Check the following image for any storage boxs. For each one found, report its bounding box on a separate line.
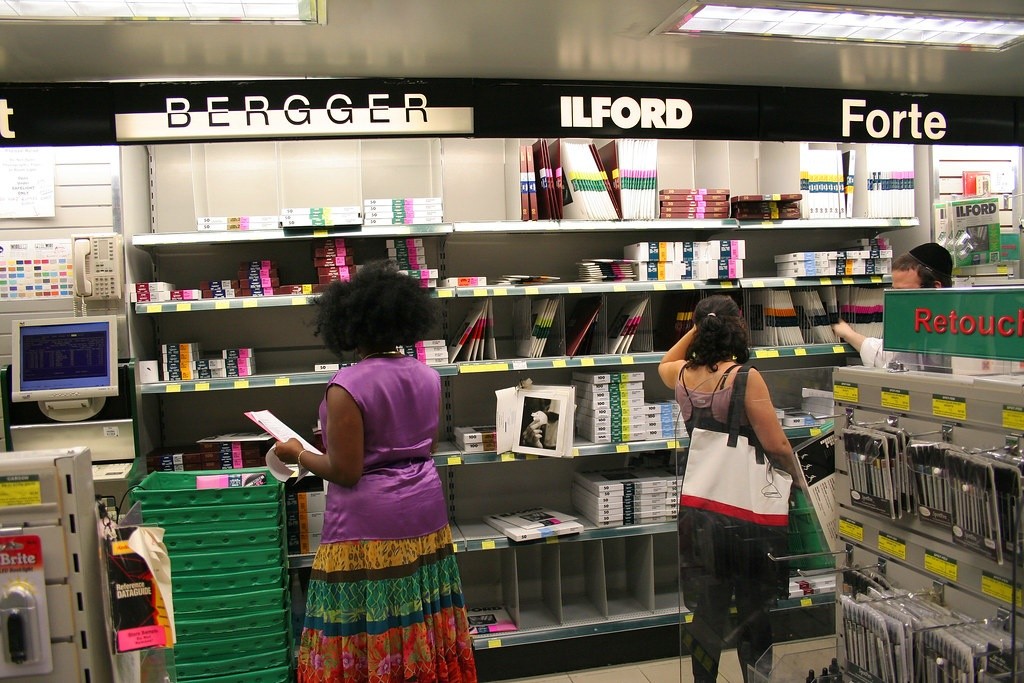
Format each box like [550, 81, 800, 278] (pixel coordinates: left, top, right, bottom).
[132, 464, 297, 682]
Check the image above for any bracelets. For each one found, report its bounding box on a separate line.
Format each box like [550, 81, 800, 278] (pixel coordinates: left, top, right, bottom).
[298, 450, 310, 468]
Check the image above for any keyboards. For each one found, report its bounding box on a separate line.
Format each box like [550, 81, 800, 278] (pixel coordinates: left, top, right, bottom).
[91, 463, 133, 479]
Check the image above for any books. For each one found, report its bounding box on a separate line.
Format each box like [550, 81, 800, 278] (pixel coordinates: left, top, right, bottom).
[448, 138, 916, 546]
[146, 432, 274, 474]
[102, 500, 178, 655]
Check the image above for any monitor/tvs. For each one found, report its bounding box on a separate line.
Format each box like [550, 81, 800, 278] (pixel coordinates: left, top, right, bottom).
[12, 315, 119, 402]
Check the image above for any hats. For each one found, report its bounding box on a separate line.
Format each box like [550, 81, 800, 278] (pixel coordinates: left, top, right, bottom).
[909, 243, 953, 277]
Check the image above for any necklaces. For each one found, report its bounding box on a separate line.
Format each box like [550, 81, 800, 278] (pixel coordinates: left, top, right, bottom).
[362, 351, 401, 360]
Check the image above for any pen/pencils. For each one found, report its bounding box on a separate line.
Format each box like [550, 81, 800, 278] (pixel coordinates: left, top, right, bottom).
[804, 564, 1024, 683]
[910, 438, 1024, 567]
[841, 420, 917, 521]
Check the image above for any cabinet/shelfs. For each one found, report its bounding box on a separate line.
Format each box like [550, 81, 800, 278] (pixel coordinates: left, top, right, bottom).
[811, 357, 1023, 683]
[116, 142, 918, 656]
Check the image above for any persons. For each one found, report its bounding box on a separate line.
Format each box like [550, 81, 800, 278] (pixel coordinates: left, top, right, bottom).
[657, 295, 811, 683]
[522, 419, 543, 448]
[272, 260, 476, 683]
[831, 241, 953, 373]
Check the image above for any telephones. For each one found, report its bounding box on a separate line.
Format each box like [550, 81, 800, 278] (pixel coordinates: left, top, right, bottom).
[70, 232, 124, 301]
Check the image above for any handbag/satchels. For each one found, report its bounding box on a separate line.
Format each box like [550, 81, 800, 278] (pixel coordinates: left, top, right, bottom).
[679, 364, 794, 527]
